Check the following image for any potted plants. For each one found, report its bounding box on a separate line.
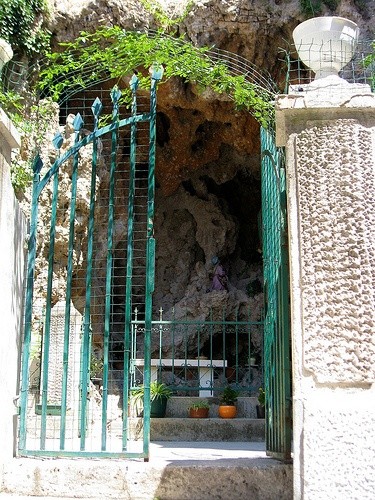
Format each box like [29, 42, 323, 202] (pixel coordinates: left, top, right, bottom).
[255, 387, 265, 419]
[246, 352, 255, 365]
[216, 385, 238, 419]
[185, 399, 211, 419]
[130, 379, 174, 418]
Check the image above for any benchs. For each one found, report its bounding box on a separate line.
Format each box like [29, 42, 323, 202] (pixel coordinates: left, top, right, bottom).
[129, 358, 228, 396]
